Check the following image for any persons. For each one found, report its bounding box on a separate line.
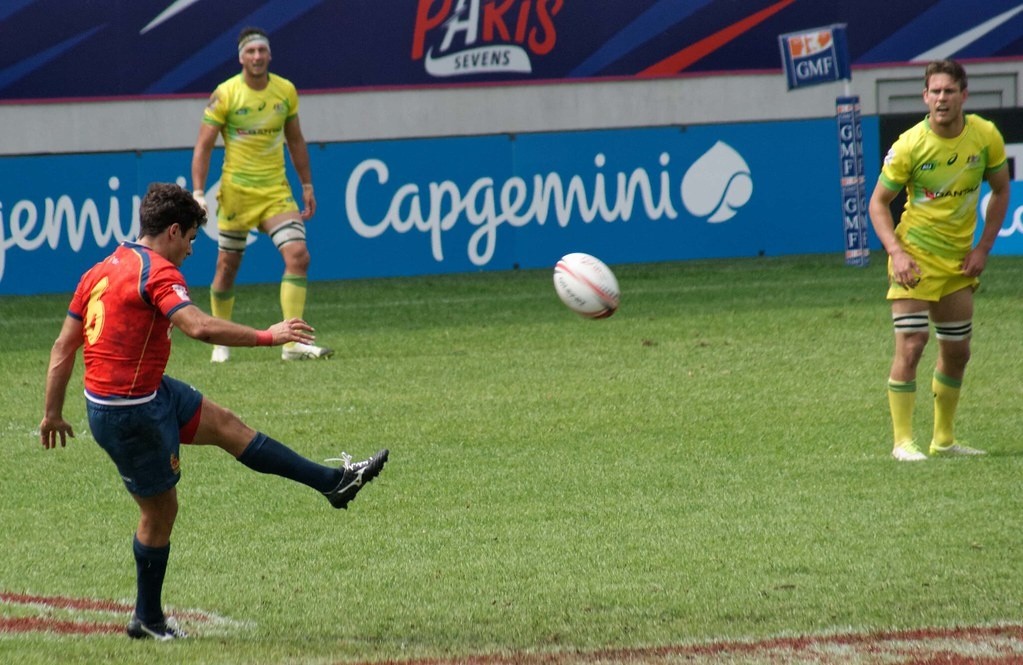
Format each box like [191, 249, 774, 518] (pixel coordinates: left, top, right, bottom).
[867, 59, 1011, 462]
[192, 26, 335, 363]
[39, 181, 392, 643]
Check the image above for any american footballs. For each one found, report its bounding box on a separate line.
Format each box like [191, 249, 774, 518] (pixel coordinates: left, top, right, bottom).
[553, 251, 620, 319]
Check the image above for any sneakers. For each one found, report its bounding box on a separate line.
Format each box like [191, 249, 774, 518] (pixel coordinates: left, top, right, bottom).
[929, 439, 986, 456]
[322, 449, 389, 511]
[893, 439, 927, 461]
[282, 342, 334, 359]
[127, 611, 188, 641]
[210, 346, 229, 362]
